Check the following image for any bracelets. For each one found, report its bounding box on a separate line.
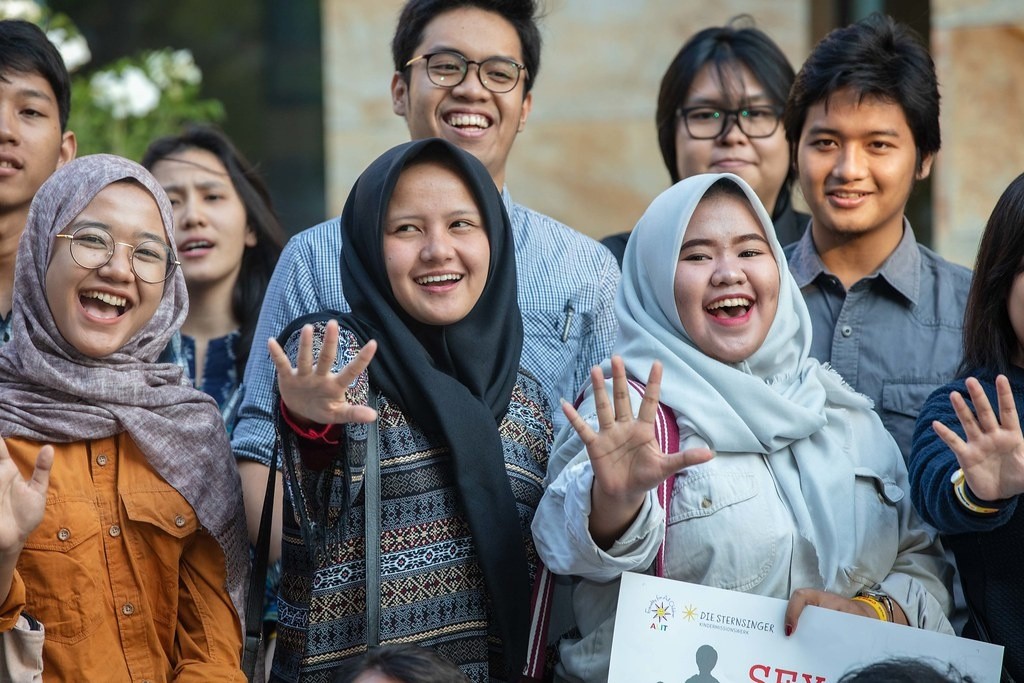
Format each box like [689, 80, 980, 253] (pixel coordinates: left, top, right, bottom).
[279, 400, 338, 445]
[852, 596, 887, 621]
[950, 468, 1000, 513]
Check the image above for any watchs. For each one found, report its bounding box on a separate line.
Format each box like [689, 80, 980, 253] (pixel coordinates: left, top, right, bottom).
[855, 589, 895, 622]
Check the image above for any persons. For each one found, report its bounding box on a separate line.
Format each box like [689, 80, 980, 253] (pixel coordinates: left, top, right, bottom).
[0, 0, 1024, 683]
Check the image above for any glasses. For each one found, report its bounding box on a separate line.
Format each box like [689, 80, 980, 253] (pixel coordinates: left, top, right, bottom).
[680, 106, 786, 140]
[403, 51, 530, 93]
[56, 225, 181, 284]
